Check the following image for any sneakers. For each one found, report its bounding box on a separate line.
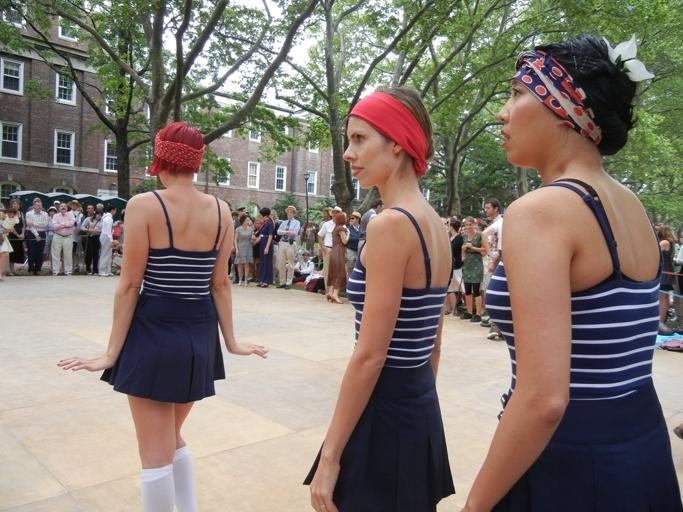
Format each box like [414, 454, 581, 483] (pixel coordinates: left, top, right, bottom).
[256, 282, 269, 288]
[86, 270, 92, 275]
[232, 279, 248, 286]
[75, 265, 80, 272]
[488, 331, 502, 339]
[277, 284, 290, 289]
[98, 271, 114, 276]
[8, 271, 21, 276]
[460, 311, 491, 327]
[29, 270, 41, 276]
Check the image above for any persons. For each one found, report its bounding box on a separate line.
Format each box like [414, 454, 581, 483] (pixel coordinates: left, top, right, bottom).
[57, 121, 268, 512]
[461, 35, 683, 510]
[440, 197, 505, 341]
[223, 200, 385, 304]
[1, 197, 126, 276]
[304, 87, 455, 511]
[653, 222, 682, 353]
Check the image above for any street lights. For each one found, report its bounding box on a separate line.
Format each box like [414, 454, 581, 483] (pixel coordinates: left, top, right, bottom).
[303, 171, 311, 223]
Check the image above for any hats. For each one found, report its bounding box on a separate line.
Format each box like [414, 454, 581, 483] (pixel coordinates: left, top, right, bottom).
[351, 211, 362, 218]
[324, 206, 334, 216]
[331, 205, 342, 215]
[285, 206, 297, 213]
[68, 200, 81, 208]
[54, 201, 60, 205]
[47, 206, 58, 213]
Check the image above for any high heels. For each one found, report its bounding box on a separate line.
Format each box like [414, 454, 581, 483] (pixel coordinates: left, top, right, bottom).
[326, 294, 343, 304]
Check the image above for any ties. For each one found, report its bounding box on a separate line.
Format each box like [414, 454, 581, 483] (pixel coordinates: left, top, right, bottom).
[284, 220, 291, 240]
